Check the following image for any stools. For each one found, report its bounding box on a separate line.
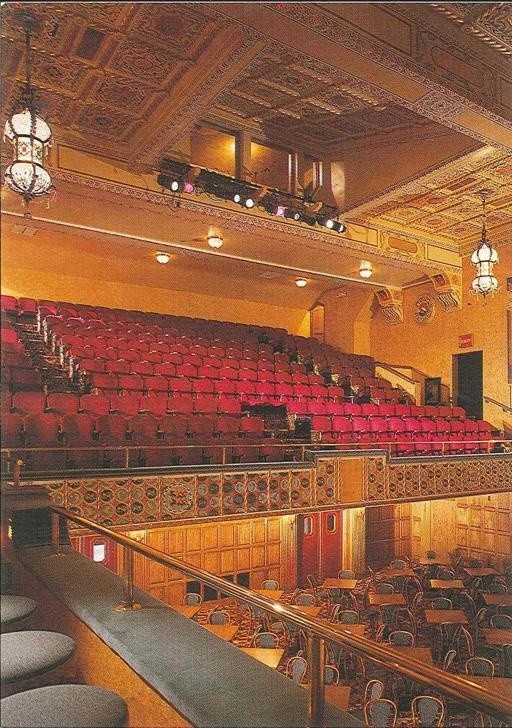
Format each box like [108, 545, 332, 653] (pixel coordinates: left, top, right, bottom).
[1, 682, 131, 728]
[2, 592, 39, 628]
[1, 629, 79, 687]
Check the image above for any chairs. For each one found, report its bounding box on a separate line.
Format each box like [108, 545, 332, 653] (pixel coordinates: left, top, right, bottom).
[164, 551, 511, 727]
[0, 288, 512, 466]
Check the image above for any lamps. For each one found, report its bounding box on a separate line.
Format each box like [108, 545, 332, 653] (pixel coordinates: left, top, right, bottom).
[155, 157, 371, 289]
[468, 185, 498, 300]
[3, 13, 54, 202]
